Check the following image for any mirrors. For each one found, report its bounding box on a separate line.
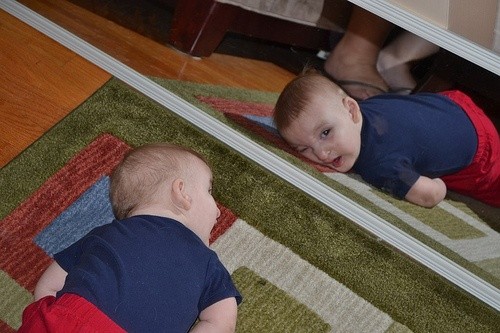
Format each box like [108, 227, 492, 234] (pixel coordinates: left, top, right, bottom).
[14, 0, 500, 293]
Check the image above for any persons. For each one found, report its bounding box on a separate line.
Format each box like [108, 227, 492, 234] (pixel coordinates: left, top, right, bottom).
[321, 5, 442, 102]
[274, 71, 499, 208]
[15, 142, 243, 333]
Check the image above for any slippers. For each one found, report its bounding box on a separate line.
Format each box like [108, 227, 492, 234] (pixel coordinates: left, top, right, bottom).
[322, 61, 392, 103]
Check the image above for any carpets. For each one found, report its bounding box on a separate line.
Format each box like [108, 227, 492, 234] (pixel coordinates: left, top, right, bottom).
[1, 76, 500, 332]
[147, 74, 499, 290]
